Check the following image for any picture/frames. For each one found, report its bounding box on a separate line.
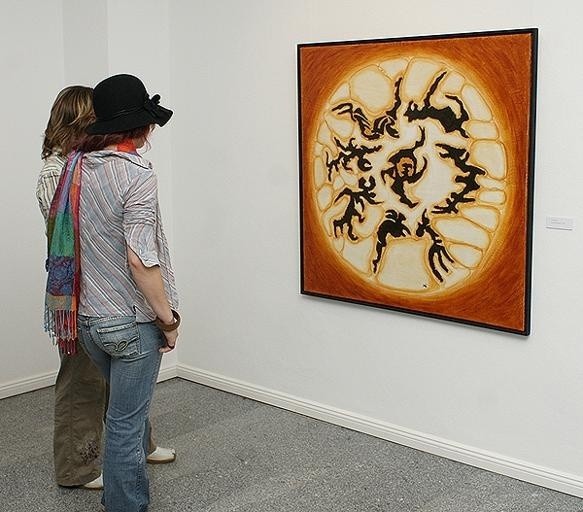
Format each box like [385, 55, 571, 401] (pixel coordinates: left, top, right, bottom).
[293, 29, 539, 341]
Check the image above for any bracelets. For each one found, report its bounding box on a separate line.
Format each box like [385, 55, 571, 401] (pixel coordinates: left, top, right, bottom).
[154, 311, 181, 332]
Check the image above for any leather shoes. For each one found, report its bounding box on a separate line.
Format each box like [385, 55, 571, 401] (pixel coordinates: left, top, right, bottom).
[81, 472, 104, 488]
[144, 445, 175, 463]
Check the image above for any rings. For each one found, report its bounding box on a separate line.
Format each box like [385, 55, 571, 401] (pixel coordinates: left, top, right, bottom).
[168, 344, 175, 349]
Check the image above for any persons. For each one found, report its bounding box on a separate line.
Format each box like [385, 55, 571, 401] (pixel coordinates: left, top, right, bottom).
[36, 85, 176, 490]
[66, 74, 181, 512]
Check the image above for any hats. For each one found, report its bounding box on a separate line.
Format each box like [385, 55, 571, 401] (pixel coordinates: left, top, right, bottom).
[85, 74, 173, 134]
